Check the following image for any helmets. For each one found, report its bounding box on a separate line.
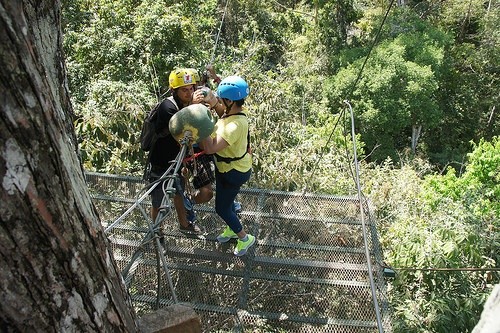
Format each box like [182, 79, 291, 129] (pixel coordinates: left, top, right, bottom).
[216, 76, 249, 100]
[169, 68, 196, 89]
[187, 68, 200, 81]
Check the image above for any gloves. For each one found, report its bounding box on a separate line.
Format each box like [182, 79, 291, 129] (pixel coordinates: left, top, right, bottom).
[201, 87, 218, 108]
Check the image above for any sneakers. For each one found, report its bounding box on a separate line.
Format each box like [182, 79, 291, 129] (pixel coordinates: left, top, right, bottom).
[216, 225, 239, 242]
[234, 234, 256, 256]
[180, 223, 205, 235]
[185, 194, 195, 221]
[159, 229, 166, 245]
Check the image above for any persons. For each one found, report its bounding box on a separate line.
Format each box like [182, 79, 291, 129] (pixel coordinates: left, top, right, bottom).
[187, 64, 222, 206]
[201, 76, 256, 256]
[143, 68, 205, 255]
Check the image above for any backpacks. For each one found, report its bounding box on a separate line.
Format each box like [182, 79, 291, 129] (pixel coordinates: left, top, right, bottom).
[139, 97, 180, 152]
